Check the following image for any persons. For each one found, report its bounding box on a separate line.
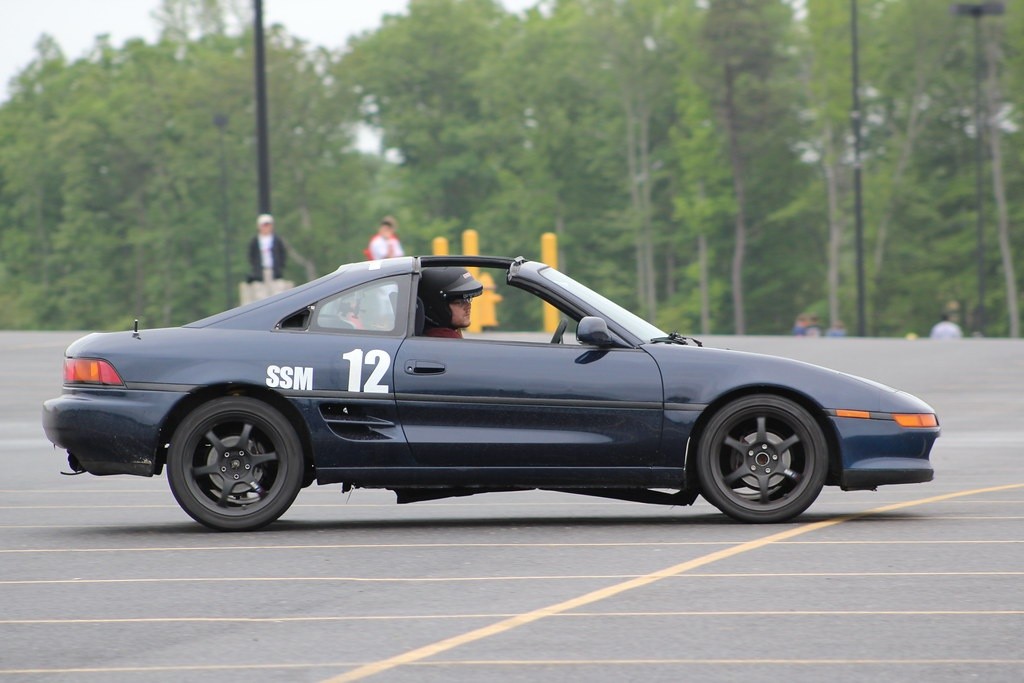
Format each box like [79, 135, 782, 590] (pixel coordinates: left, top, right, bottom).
[930, 312, 961, 340]
[793, 313, 848, 337]
[417, 267, 483, 338]
[365, 215, 405, 260]
[243, 214, 286, 300]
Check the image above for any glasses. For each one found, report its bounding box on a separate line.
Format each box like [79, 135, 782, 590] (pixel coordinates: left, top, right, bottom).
[450, 298, 473, 305]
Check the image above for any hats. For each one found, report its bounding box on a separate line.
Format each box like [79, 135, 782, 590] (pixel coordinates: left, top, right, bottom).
[257, 214, 273, 224]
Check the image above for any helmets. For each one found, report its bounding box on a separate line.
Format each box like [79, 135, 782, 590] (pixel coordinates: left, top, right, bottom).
[417, 266, 483, 328]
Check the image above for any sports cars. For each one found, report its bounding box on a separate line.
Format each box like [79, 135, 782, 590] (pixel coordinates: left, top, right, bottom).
[40, 254, 943, 535]
